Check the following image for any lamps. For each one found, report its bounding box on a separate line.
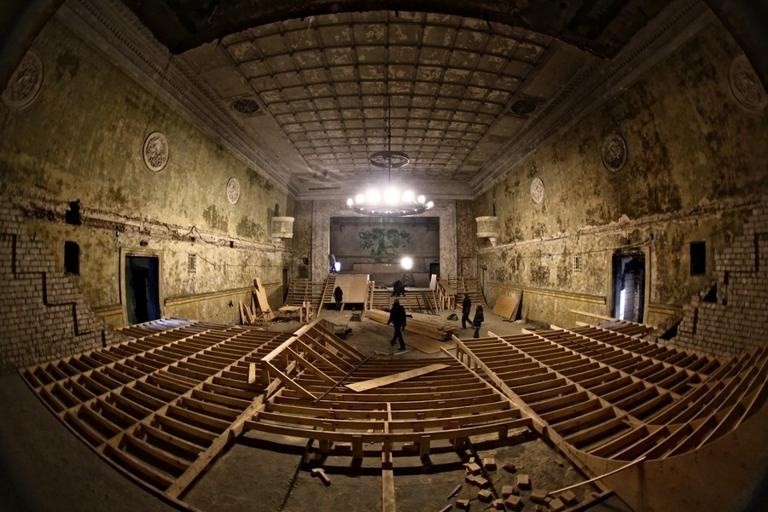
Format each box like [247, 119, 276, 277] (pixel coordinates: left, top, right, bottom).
[347, 97, 434, 218]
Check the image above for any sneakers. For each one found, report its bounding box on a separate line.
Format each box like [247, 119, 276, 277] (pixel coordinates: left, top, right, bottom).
[397, 348, 406, 350]
[390, 340, 396, 343]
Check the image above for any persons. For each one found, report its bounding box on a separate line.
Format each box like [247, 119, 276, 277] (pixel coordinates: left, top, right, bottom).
[391, 279, 406, 297]
[462, 292, 484, 338]
[328, 253, 336, 275]
[333, 286, 343, 311]
[386, 298, 407, 351]
